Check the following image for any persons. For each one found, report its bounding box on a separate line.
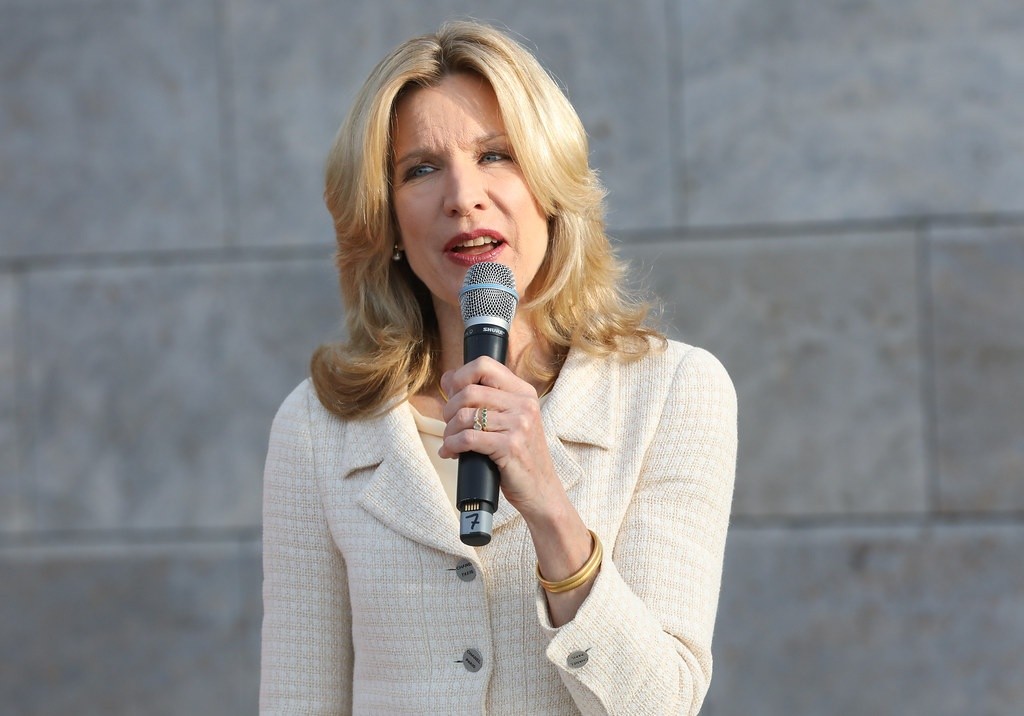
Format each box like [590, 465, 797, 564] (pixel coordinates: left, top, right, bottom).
[261, 23, 738, 716]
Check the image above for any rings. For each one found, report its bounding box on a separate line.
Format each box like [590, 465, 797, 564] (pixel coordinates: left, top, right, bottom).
[482, 408, 487, 431]
[474, 408, 482, 431]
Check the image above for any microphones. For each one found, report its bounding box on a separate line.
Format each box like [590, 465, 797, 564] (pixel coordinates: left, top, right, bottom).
[458, 263, 519, 549]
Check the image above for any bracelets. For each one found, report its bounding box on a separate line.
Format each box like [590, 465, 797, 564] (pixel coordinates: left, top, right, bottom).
[536, 532, 603, 594]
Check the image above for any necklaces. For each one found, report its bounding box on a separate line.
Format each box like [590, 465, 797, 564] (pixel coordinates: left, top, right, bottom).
[437, 381, 555, 403]
